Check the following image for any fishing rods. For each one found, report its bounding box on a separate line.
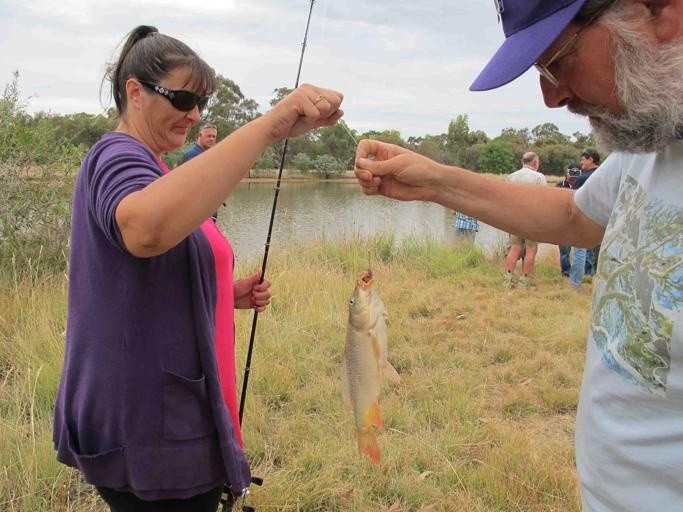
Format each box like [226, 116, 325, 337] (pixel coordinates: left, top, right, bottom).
[220, 0, 376, 512]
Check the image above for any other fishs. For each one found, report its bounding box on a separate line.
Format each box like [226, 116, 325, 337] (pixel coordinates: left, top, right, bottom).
[343, 268, 402, 468]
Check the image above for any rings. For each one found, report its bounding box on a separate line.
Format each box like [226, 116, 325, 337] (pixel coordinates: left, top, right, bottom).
[313, 94, 324, 106]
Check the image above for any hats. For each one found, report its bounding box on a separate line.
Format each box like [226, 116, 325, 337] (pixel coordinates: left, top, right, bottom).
[469, 1, 584, 91]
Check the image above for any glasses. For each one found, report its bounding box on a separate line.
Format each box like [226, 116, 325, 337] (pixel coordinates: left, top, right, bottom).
[136, 80, 209, 113]
[533, 1, 611, 86]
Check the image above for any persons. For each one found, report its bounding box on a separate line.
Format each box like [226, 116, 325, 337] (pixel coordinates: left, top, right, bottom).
[46, 24, 346, 510]
[499, 151, 550, 294]
[181, 123, 218, 226]
[565, 149, 604, 288]
[352, 1, 681, 512]
[447, 207, 479, 246]
[552, 162, 593, 280]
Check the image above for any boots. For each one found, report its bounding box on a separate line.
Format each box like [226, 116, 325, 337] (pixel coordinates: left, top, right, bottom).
[502, 273, 514, 289]
[518, 276, 537, 291]
